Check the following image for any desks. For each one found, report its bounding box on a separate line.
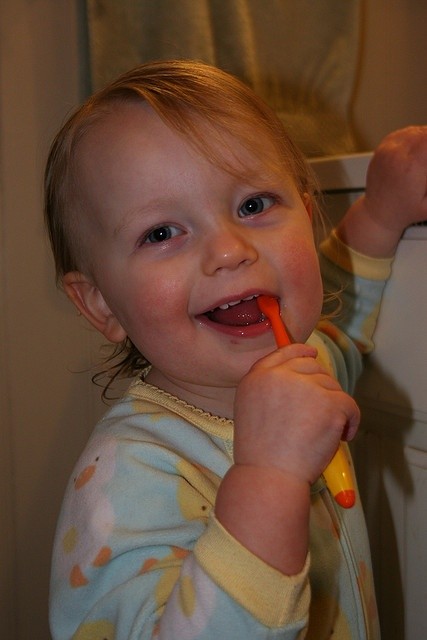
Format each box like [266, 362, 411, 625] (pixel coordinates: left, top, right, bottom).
[287, 149, 425, 639]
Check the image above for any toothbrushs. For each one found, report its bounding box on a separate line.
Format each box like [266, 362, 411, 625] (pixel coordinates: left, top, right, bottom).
[257, 294, 356, 508]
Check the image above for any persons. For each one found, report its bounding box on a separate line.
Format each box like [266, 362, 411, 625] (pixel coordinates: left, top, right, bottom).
[44, 57, 427, 639]
